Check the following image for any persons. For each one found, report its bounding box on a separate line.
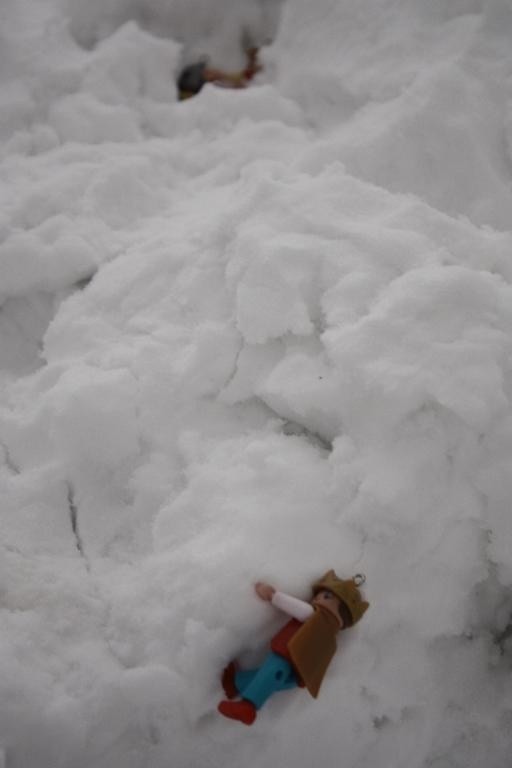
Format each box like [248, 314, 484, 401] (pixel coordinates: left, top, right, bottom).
[213, 568, 371, 726]
